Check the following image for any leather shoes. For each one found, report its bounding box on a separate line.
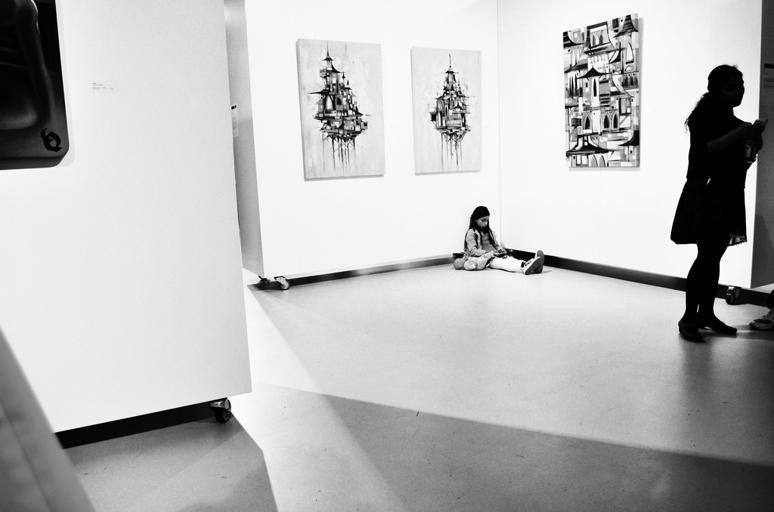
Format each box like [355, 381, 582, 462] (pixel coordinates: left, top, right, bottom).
[678, 313, 737, 342]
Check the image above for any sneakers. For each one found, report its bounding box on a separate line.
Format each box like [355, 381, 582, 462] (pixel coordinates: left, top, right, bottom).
[522, 250, 544, 275]
[748, 311, 774, 332]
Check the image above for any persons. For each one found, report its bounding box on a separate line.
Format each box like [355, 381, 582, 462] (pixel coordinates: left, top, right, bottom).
[462, 206, 545, 275]
[748, 286, 774, 333]
[667, 63, 766, 342]
